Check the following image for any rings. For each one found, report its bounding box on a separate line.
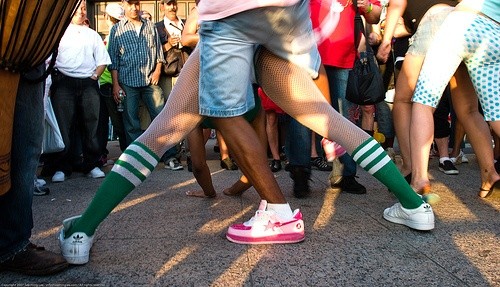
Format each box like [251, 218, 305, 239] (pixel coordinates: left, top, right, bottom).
[361, 0, 364, 4]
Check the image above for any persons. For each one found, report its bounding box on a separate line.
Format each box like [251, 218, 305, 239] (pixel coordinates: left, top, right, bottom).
[58, 41, 436, 259]
[32, 0, 500, 204]
[0, 63, 70, 275]
[195, 0, 347, 246]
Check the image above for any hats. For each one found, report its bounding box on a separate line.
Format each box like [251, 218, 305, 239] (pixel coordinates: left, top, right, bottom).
[162, 0, 177, 4]
[106, 3, 124, 20]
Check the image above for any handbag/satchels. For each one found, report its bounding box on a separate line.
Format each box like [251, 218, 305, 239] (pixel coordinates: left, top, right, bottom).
[40, 97, 65, 154]
[163, 46, 185, 78]
[345, 49, 386, 105]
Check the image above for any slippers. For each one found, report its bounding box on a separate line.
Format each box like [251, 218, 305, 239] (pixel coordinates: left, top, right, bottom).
[478, 180, 499, 198]
[387, 173, 412, 192]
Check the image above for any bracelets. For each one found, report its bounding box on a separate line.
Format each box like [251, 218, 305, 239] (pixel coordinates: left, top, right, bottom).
[364, 2, 372, 13]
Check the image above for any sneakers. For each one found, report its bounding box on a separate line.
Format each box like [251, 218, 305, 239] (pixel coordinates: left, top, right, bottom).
[34, 178, 46, 185]
[292, 174, 309, 197]
[321, 139, 346, 161]
[34, 183, 49, 195]
[461, 153, 468, 162]
[285, 163, 291, 171]
[310, 156, 333, 171]
[59, 214, 98, 264]
[163, 159, 182, 171]
[220, 157, 238, 171]
[87, 167, 105, 178]
[187, 156, 193, 172]
[330, 172, 367, 195]
[52, 171, 65, 181]
[225, 198, 306, 244]
[384, 201, 435, 232]
[270, 158, 282, 172]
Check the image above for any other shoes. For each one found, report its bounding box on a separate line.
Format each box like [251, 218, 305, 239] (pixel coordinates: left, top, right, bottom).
[0, 243, 68, 276]
[429, 149, 439, 159]
[214, 145, 220, 152]
[439, 159, 459, 175]
